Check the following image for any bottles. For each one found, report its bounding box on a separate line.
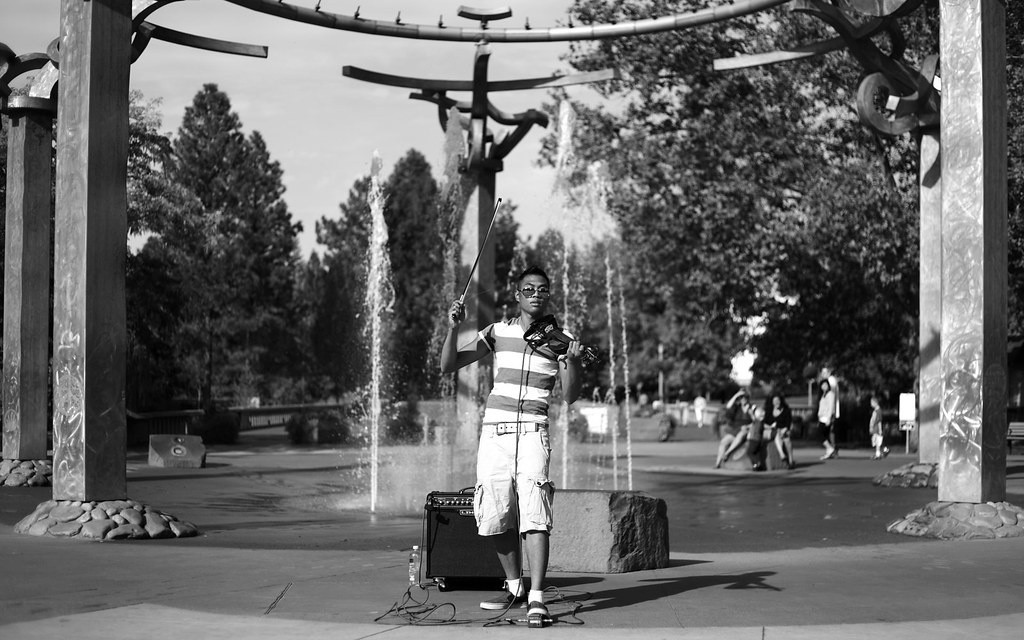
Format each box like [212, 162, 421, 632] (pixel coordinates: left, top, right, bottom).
[408, 545, 421, 586]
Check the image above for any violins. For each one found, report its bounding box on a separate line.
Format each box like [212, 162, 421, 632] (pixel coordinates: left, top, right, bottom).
[524, 314, 606, 368]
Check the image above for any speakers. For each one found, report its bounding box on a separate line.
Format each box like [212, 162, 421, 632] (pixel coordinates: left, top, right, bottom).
[426, 490, 509, 579]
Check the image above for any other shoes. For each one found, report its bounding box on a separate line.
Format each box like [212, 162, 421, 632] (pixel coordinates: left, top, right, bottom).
[820, 448, 835, 460]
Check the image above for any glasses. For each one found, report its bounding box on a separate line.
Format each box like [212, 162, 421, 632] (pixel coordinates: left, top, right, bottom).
[516, 287, 549, 299]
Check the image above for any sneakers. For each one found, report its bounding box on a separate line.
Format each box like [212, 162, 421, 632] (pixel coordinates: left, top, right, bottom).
[526, 601, 549, 620]
[479, 591, 528, 609]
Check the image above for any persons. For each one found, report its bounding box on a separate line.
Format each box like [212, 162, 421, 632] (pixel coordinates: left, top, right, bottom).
[440, 266, 583, 628]
[633, 363, 891, 473]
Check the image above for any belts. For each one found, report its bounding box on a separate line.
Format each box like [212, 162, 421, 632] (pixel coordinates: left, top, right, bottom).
[495, 423, 539, 435]
